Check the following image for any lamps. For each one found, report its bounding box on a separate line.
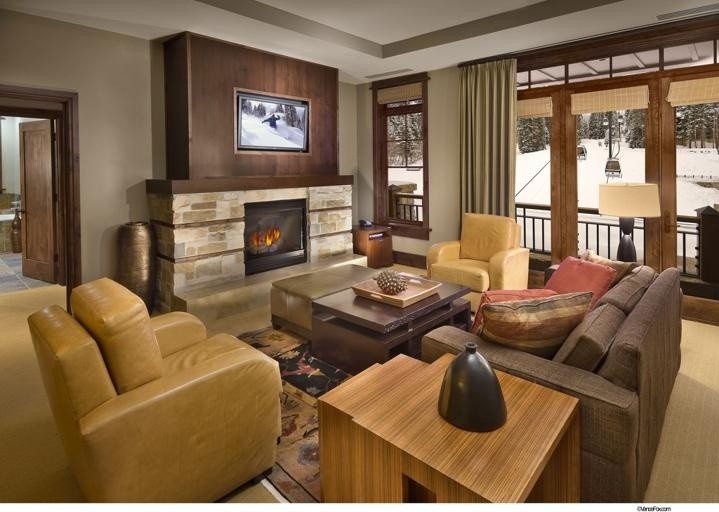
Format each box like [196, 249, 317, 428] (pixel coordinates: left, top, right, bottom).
[598, 183, 661, 262]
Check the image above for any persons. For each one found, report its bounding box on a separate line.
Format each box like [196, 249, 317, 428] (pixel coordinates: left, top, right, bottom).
[261, 114, 281, 130]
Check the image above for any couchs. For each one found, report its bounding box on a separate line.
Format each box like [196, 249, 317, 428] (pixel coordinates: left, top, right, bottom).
[419, 263, 684, 503]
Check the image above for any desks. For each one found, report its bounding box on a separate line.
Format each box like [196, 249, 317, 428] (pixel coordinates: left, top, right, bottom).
[353, 224, 393, 268]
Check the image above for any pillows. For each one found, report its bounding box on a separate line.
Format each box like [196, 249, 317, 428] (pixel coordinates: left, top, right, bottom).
[470, 255, 660, 372]
[459, 213, 516, 262]
[70, 276, 165, 396]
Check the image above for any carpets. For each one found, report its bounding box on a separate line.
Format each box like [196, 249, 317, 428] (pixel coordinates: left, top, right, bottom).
[234, 325, 356, 502]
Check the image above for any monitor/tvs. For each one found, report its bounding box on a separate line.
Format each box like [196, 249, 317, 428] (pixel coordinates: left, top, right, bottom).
[237, 94, 309, 151]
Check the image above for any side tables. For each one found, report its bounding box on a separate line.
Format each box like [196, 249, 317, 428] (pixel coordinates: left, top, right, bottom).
[317, 351, 582, 502]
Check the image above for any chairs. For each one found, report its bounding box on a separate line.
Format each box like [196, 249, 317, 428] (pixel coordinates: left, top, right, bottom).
[26, 279, 283, 502]
[425, 211, 530, 315]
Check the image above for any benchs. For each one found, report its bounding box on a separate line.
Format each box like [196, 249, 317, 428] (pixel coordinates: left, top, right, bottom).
[270, 264, 383, 342]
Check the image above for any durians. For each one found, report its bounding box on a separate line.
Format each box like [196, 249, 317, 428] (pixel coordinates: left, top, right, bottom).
[376, 270, 408, 294]
